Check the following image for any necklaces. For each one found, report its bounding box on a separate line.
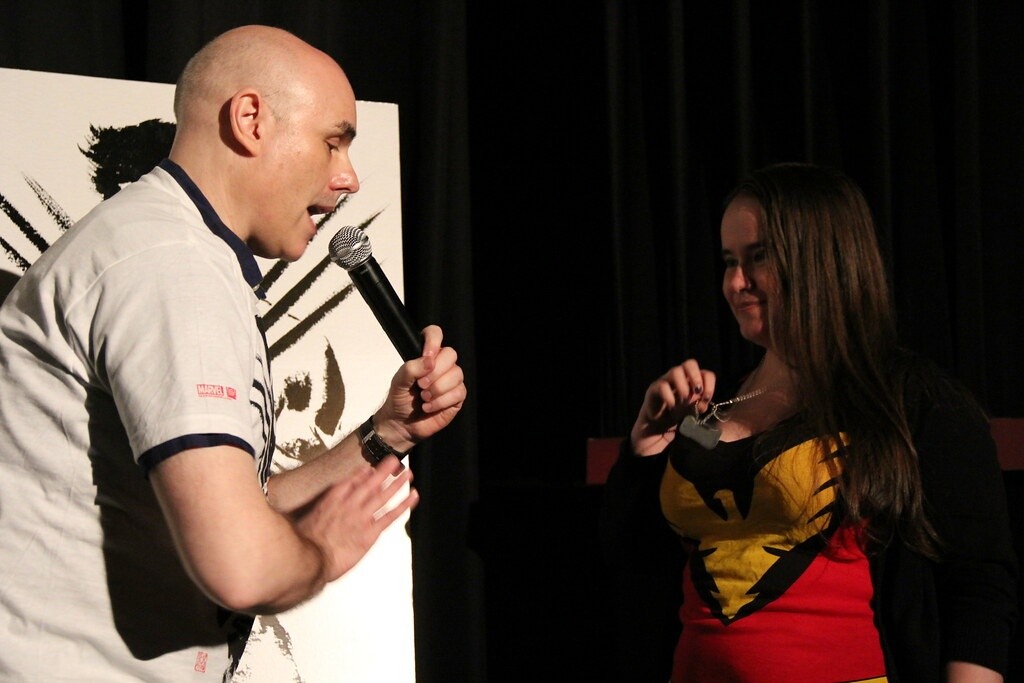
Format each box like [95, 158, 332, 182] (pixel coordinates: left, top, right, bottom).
[679, 354, 779, 449]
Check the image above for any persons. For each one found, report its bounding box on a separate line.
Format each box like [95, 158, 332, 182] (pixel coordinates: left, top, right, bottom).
[0, 25, 466, 683]
[604, 162, 1001, 683]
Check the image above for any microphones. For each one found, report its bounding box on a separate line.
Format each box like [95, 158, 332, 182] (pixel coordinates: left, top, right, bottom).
[328, 227, 425, 363]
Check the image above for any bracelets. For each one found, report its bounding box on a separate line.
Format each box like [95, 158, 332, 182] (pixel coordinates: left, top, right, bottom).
[358, 414, 411, 477]
